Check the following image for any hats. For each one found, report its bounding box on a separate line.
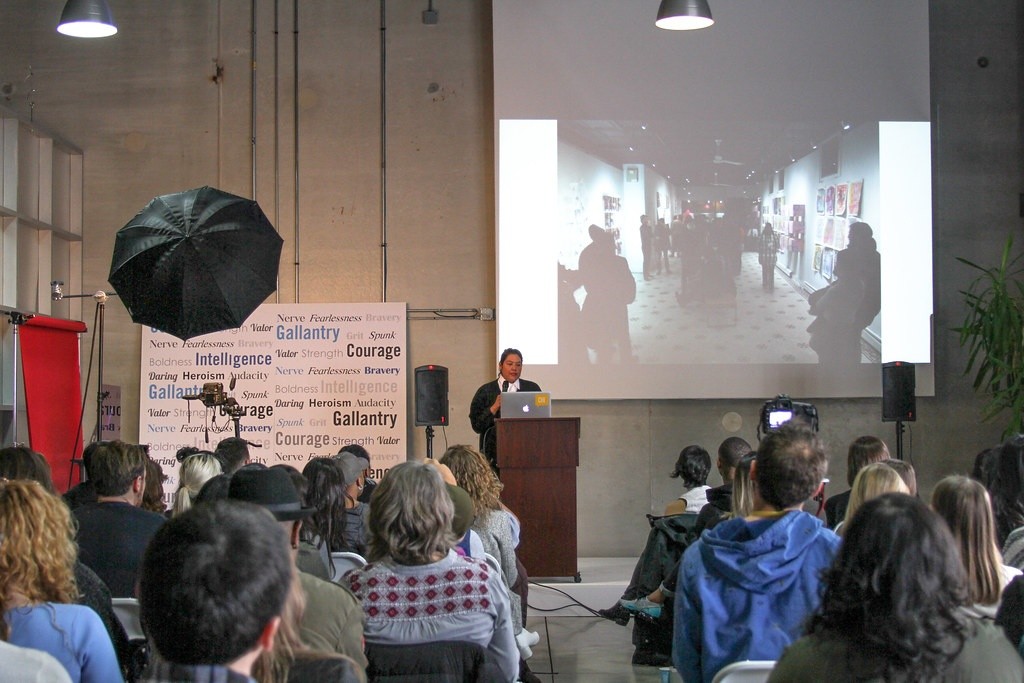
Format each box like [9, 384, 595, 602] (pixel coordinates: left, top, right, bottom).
[227, 466, 319, 522]
[331, 451, 368, 485]
[658, 217, 665, 222]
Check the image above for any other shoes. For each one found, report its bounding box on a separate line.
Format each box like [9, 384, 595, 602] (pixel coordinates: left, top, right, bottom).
[666, 269, 672, 272]
[644, 276, 651, 280]
[657, 270, 661, 274]
[599, 601, 630, 626]
[620, 598, 666, 623]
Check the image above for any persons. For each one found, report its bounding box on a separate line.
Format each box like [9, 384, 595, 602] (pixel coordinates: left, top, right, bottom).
[133, 437, 379, 683]
[338, 445, 542, 683]
[599, 416, 1024, 683]
[468, 348, 541, 480]
[558, 213, 881, 362]
[0, 448, 128, 683]
[60, 441, 169, 598]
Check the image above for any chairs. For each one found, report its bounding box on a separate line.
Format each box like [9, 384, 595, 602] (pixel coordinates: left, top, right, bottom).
[631, 514, 700, 668]
[366, 639, 510, 683]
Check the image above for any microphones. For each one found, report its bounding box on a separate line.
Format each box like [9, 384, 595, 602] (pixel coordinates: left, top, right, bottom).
[502, 381, 509, 393]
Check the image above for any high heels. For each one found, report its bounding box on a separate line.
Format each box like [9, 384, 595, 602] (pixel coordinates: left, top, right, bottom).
[515, 628, 539, 660]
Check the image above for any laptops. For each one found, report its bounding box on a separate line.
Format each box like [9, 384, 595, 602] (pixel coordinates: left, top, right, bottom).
[501, 391, 551, 417]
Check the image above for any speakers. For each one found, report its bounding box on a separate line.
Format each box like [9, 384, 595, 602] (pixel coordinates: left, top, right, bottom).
[414, 365, 450, 426]
[882, 361, 917, 421]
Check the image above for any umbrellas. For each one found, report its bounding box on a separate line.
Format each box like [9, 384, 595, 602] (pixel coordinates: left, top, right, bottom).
[108, 184, 284, 341]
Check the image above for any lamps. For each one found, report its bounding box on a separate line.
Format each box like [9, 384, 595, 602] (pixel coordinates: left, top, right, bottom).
[655, 0, 715, 31]
[56, 0, 118, 38]
